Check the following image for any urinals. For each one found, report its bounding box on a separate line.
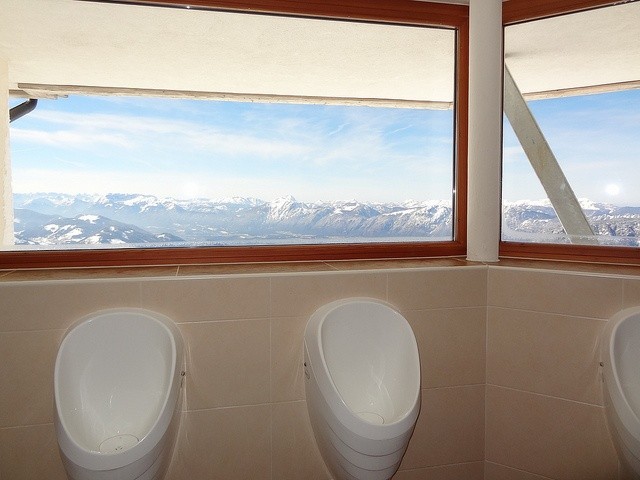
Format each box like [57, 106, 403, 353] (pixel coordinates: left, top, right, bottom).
[50, 308, 184, 477]
[600, 306, 639, 475]
[303, 296, 424, 479]
